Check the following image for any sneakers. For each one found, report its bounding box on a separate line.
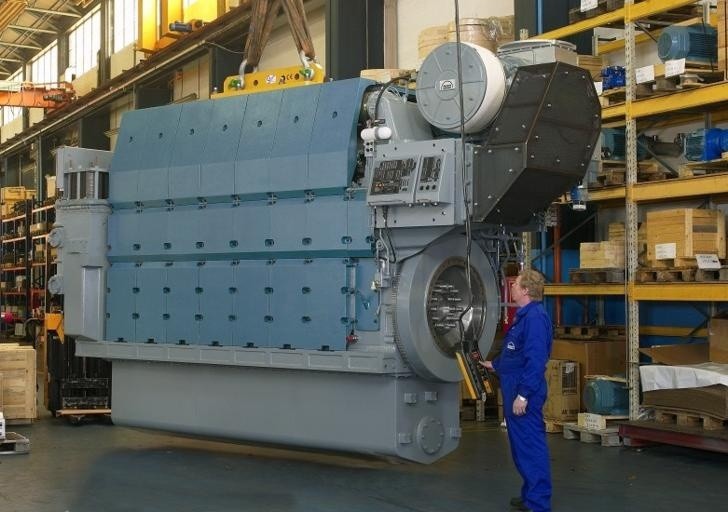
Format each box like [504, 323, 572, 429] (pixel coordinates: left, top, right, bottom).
[511, 498, 554, 511]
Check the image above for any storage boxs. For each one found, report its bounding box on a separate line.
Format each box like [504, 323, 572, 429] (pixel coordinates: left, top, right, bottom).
[0, 344, 38, 420]
[503, 208, 727, 421]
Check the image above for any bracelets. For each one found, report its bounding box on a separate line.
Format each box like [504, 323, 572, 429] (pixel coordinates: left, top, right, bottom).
[518, 393, 527, 401]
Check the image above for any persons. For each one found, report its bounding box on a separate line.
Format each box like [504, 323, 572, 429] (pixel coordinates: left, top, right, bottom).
[478, 269, 556, 512]
